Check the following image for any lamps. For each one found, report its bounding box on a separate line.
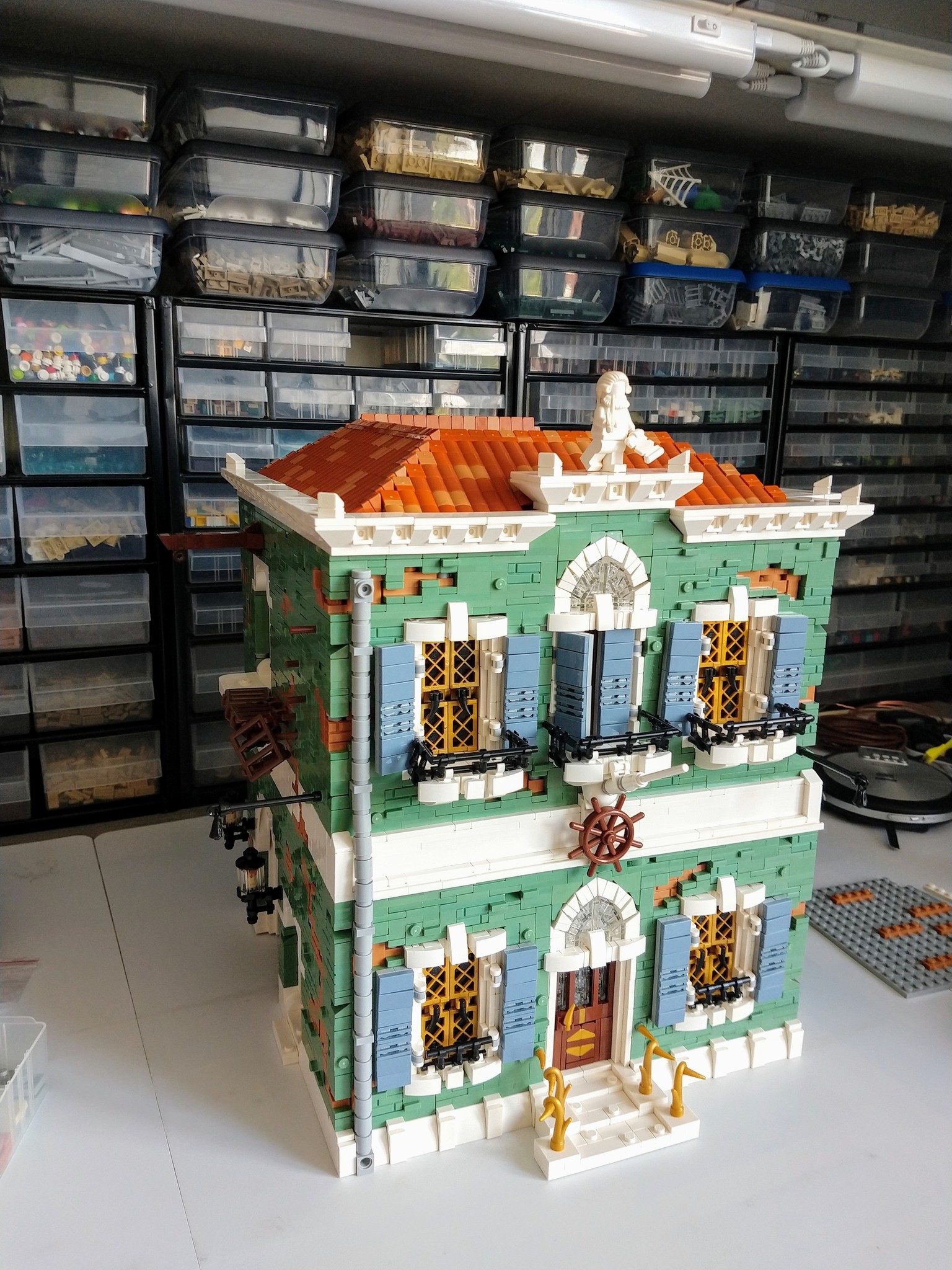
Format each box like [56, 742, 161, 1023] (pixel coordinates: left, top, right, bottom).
[170, 1, 952, 148]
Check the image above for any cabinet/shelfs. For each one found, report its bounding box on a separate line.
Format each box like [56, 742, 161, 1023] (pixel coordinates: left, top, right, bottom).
[3, 71, 950, 839]
